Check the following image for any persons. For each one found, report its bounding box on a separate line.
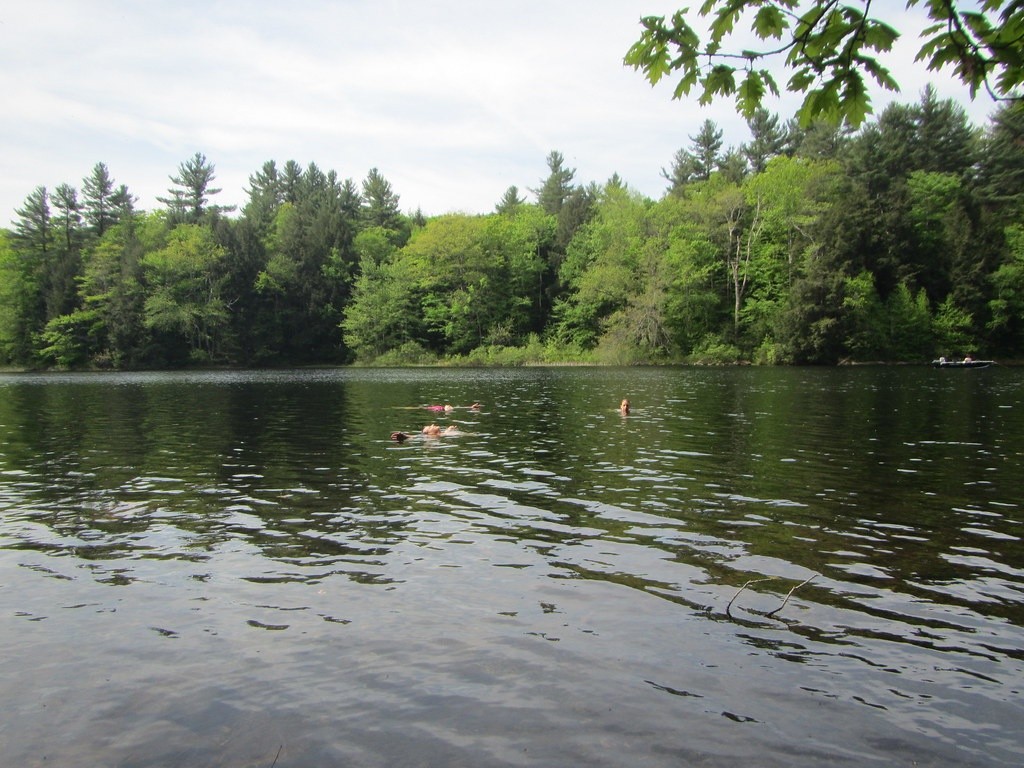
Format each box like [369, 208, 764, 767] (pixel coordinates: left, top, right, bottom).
[621, 399, 630, 416]
[391, 424, 458, 441]
[444, 402, 480, 412]
